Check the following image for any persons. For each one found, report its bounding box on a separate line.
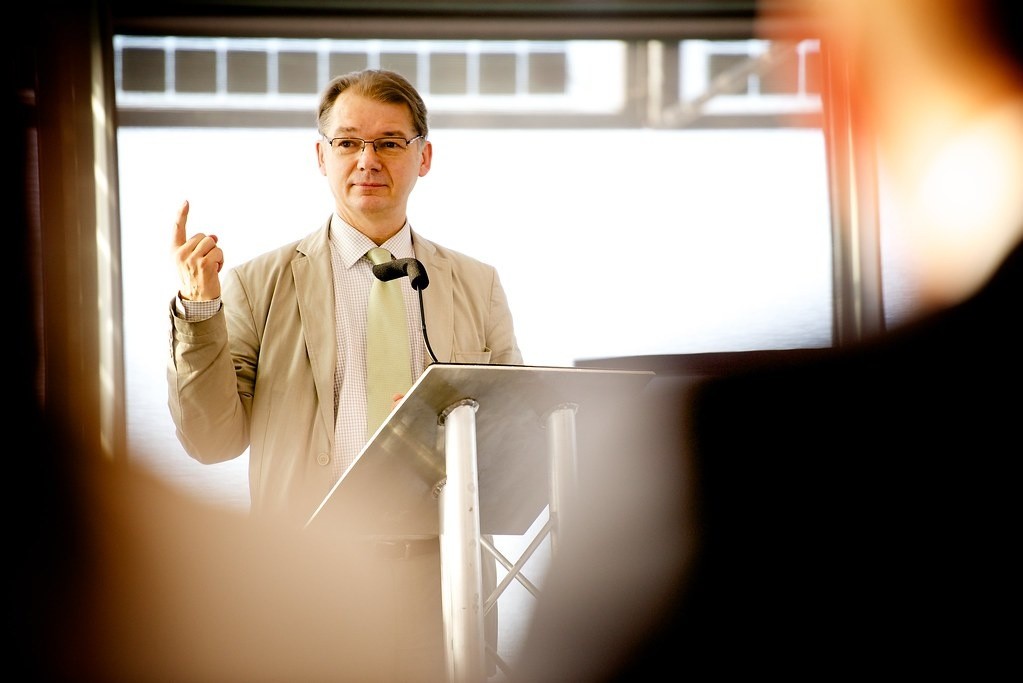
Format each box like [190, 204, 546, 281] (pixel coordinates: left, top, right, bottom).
[166, 68, 525, 683]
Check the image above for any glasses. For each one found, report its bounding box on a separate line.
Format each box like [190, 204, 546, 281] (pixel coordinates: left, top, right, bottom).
[323, 134, 423, 156]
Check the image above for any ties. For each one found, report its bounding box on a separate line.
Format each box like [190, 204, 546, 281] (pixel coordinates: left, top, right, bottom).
[367, 248, 412, 441]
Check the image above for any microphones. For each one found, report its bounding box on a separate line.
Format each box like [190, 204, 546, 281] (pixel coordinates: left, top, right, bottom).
[372, 257, 438, 362]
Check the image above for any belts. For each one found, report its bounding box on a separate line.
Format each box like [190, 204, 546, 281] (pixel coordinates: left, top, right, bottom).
[348, 540, 439, 559]
